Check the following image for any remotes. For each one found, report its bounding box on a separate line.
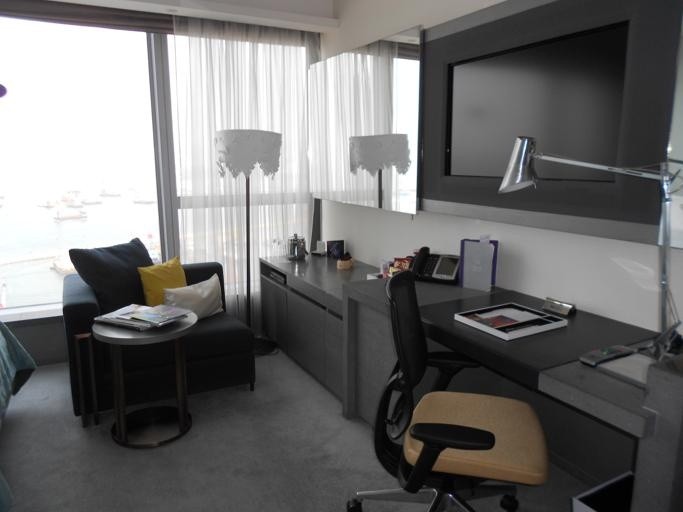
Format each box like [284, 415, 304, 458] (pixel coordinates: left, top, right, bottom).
[579, 345, 635, 366]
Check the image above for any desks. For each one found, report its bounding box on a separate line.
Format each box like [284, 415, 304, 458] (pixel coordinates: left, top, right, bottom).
[257, 250, 381, 405]
[90, 303, 201, 451]
[341, 277, 682, 512]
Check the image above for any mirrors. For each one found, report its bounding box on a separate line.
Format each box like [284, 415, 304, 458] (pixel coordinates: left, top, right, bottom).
[305, 23, 423, 217]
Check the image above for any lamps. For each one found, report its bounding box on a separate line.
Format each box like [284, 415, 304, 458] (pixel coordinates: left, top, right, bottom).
[210, 127, 285, 358]
[496, 135, 682, 363]
[348, 132, 411, 209]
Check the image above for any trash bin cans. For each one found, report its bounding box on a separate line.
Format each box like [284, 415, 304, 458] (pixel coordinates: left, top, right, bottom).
[571, 471, 636, 512]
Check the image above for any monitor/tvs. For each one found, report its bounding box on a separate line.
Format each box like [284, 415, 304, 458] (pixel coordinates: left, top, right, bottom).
[443, 21, 629, 182]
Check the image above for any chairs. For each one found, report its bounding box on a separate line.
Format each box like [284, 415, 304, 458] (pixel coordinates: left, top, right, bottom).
[62, 260, 257, 429]
[344, 267, 550, 511]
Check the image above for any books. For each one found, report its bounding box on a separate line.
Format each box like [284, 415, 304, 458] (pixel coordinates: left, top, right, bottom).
[90, 302, 192, 332]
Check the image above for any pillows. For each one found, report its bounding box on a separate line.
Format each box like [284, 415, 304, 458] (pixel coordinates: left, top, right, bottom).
[162, 271, 226, 320]
[67, 236, 156, 315]
[136, 255, 188, 307]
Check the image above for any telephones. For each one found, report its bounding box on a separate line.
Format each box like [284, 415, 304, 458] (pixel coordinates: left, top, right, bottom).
[409, 247, 461, 286]
